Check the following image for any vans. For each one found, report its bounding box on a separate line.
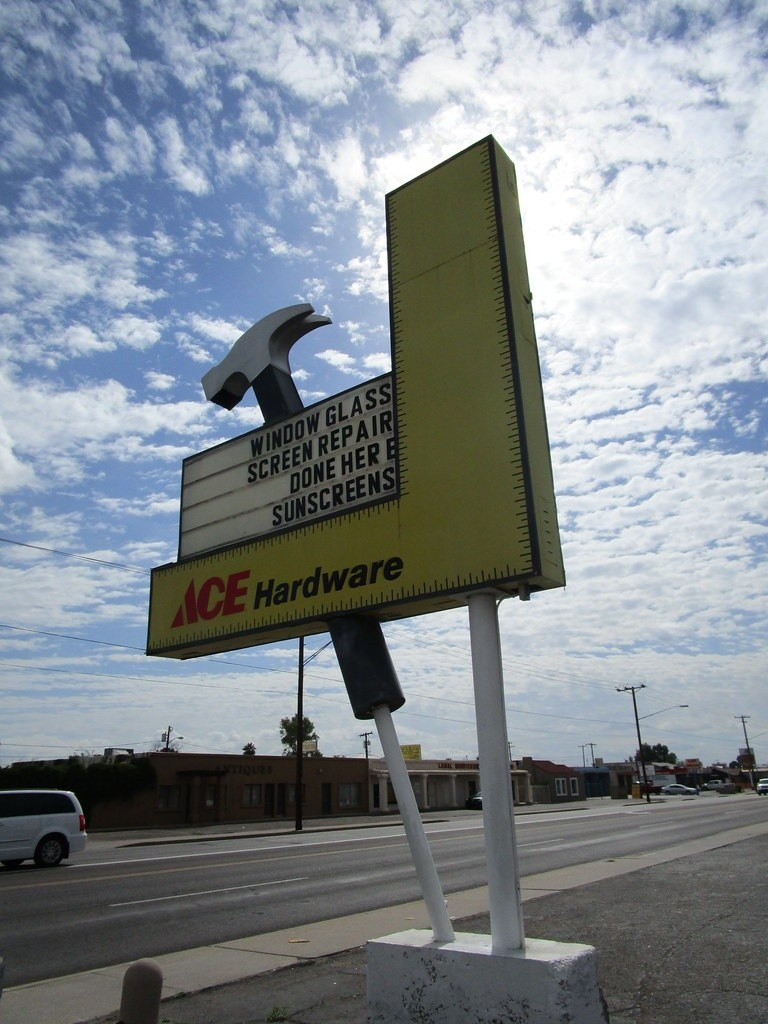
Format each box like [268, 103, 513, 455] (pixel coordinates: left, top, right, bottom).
[0, 789, 89, 868]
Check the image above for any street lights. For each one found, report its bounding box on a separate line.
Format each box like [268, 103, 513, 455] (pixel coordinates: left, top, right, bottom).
[161, 725, 184, 752]
[616, 683, 689, 804]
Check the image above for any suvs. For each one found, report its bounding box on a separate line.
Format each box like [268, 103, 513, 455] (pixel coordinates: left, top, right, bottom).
[756, 778, 768, 796]
[631, 781, 664, 796]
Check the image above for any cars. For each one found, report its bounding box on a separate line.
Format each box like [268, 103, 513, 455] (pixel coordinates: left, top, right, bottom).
[464, 786, 483, 810]
[661, 784, 697, 795]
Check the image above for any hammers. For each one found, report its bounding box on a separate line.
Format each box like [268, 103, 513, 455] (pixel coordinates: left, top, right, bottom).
[203, 303, 406, 716]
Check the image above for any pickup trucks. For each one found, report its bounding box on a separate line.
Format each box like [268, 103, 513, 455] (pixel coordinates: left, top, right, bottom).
[701, 779, 736, 791]
